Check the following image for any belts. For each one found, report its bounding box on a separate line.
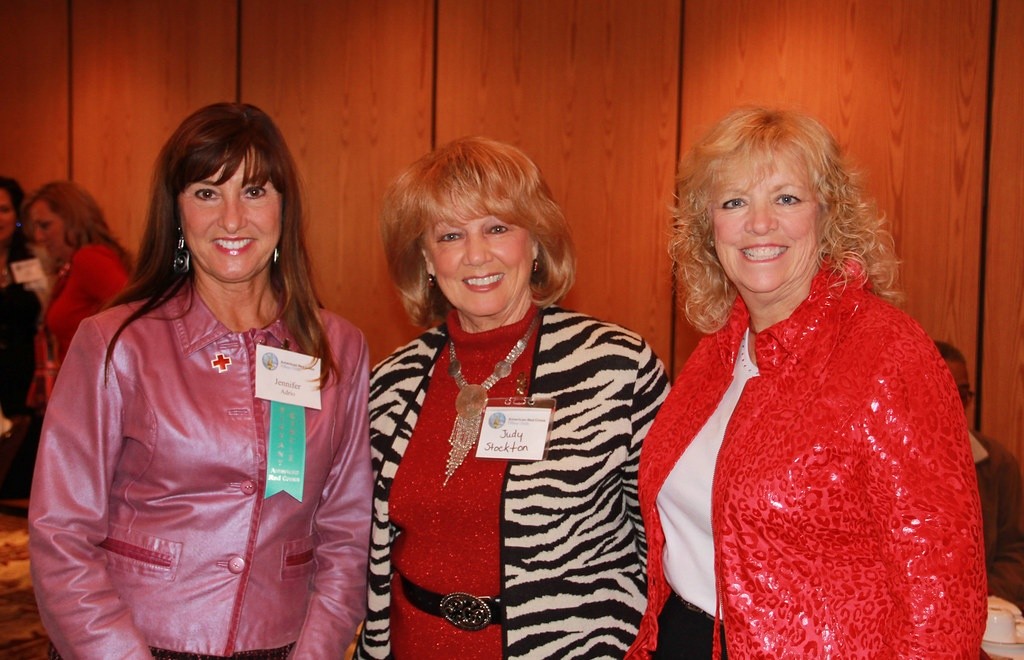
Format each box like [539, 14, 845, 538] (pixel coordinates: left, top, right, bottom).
[390, 564, 502, 631]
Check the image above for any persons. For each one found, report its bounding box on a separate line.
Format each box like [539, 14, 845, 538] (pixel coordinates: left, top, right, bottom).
[0, 174, 47, 505]
[28, 180, 135, 413]
[934, 341, 1024, 617]
[624, 106, 991, 659]
[347, 136, 673, 660]
[27, 100, 374, 660]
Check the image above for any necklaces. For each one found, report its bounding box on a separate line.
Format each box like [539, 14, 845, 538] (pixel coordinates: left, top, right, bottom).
[443, 314, 541, 488]
